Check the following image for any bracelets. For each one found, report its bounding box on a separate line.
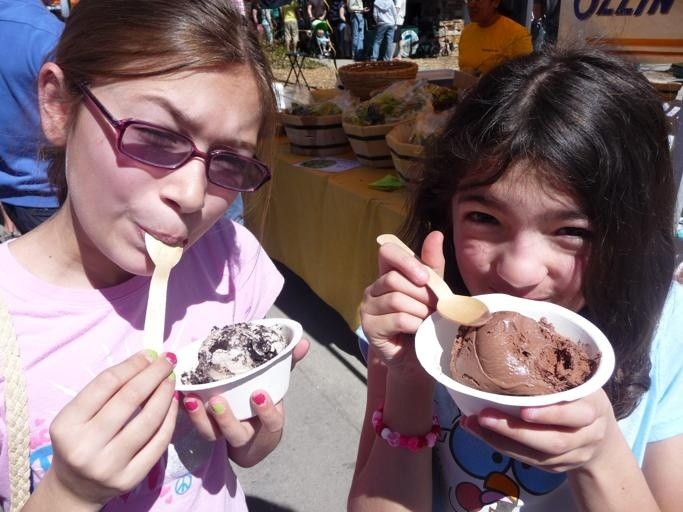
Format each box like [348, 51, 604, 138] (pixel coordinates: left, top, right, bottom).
[371, 400, 444, 454]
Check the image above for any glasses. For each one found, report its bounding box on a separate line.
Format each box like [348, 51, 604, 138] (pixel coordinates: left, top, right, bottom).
[72, 76, 271, 193]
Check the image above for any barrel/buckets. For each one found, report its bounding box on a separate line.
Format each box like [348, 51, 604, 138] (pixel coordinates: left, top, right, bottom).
[386, 122, 431, 187]
[282, 111, 347, 156]
[342, 110, 414, 168]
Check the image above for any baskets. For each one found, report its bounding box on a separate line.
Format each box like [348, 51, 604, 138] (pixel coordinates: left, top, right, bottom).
[338, 60, 418, 100]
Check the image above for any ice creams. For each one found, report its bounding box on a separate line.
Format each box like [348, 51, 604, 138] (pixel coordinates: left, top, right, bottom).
[449, 310, 600, 397]
[181, 322, 288, 385]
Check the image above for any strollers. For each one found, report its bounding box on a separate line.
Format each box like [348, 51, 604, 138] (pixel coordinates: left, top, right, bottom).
[309, 17, 336, 58]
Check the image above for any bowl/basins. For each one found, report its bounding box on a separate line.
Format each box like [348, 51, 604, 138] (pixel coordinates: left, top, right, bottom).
[170, 318, 305, 421]
[413, 294, 616, 418]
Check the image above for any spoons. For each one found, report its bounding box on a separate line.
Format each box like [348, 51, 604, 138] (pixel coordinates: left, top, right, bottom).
[144, 228, 185, 357]
[377, 233, 493, 328]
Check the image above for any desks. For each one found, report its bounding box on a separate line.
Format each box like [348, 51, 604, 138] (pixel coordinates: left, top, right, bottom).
[240, 133, 422, 340]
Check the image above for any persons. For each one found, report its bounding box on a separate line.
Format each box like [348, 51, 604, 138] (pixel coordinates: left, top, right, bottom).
[231, 0, 407, 64]
[529, 0, 554, 52]
[458, 0, 535, 80]
[1, 1, 68, 235]
[346, 44, 683, 512]
[0, 0, 311, 512]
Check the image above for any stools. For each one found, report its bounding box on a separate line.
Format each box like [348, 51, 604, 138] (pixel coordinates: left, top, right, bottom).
[283, 51, 310, 89]
